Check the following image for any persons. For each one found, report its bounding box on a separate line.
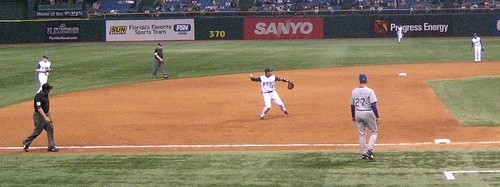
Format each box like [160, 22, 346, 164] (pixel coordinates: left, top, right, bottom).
[22, 83, 59, 152]
[153, 43, 167, 79]
[36, 56, 52, 94]
[146, 0, 497, 13]
[350, 73, 380, 158]
[471, 34, 484, 62]
[395, 26, 407, 43]
[250, 67, 294, 118]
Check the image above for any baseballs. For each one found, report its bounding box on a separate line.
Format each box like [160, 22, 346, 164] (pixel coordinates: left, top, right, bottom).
[250, 75, 253, 78]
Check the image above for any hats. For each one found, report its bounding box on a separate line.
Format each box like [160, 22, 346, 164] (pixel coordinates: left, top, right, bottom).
[359, 74, 367, 83]
[43, 55, 49, 59]
[42, 83, 53, 90]
[265, 67, 273, 71]
[473, 33, 476, 36]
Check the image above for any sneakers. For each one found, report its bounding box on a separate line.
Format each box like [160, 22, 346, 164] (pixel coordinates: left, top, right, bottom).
[162, 75, 167, 78]
[158, 43, 162, 46]
[48, 147, 58, 152]
[260, 117, 264, 120]
[23, 141, 29, 153]
[361, 154, 367, 160]
[153, 75, 157, 79]
[284, 112, 288, 116]
[365, 151, 374, 160]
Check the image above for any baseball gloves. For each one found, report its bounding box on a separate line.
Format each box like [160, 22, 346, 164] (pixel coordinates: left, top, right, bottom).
[288, 81, 294, 90]
